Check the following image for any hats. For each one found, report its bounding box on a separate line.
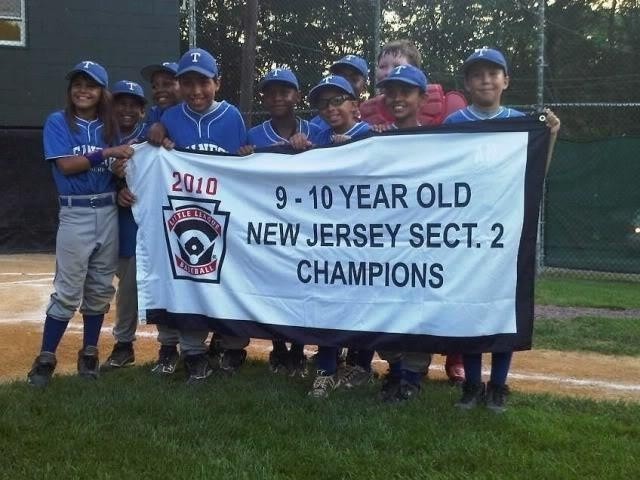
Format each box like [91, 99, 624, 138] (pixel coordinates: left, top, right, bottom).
[141, 62, 178, 84]
[111, 80, 148, 106]
[262, 69, 299, 93]
[464, 48, 507, 76]
[376, 64, 426, 94]
[309, 76, 357, 103]
[328, 54, 368, 81]
[175, 48, 218, 80]
[65, 61, 108, 90]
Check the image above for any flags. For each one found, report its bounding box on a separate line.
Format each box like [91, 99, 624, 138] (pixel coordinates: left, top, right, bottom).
[121, 112, 551, 355]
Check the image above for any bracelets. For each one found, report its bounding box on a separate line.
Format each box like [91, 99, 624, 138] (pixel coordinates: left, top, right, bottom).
[83, 148, 106, 168]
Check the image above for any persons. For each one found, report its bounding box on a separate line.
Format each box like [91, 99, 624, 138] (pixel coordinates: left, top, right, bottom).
[445, 48, 561, 412]
[109, 38, 471, 401]
[26, 60, 134, 388]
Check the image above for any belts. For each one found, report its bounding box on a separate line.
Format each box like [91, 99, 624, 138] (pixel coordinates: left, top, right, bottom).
[58, 195, 116, 208]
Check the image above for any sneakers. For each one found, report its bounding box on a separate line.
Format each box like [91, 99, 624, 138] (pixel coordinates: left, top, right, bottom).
[376, 373, 403, 401]
[26, 354, 57, 388]
[207, 337, 247, 371]
[486, 383, 510, 413]
[288, 353, 305, 378]
[399, 378, 420, 400]
[77, 349, 100, 381]
[101, 344, 136, 372]
[446, 356, 466, 387]
[185, 361, 214, 386]
[150, 351, 179, 377]
[270, 349, 287, 375]
[306, 350, 375, 400]
[455, 383, 485, 410]
